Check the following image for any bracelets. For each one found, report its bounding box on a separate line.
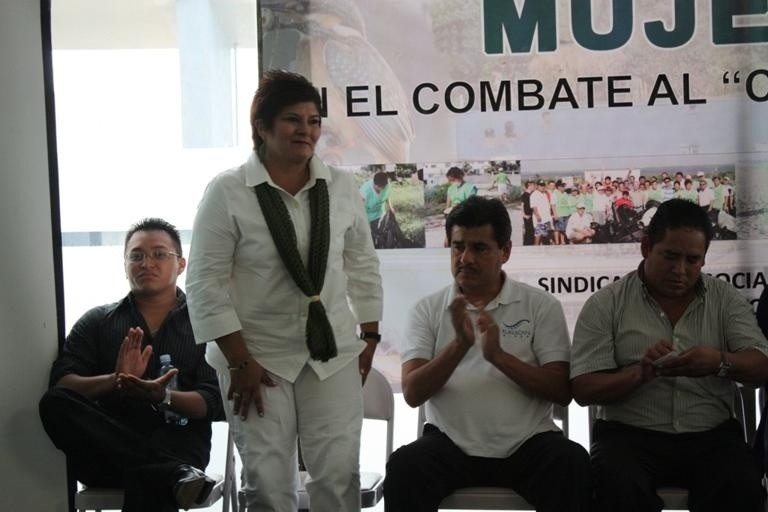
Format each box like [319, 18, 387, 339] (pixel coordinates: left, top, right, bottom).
[359, 332, 381, 343]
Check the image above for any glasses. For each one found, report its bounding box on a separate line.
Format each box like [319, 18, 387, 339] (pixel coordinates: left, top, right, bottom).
[128, 251, 179, 260]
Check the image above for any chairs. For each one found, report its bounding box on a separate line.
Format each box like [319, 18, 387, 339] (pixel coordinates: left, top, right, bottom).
[417, 401, 568, 510]
[588, 384, 748, 509]
[73, 425, 236, 512]
[238, 366, 395, 512]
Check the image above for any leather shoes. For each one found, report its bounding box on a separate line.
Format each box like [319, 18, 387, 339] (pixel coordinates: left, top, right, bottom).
[171, 463, 215, 508]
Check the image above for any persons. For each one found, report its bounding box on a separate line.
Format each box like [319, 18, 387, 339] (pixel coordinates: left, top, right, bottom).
[184, 70, 384, 512]
[445, 167, 478, 248]
[382, 197, 590, 511]
[755, 285, 768, 484]
[39, 220, 225, 512]
[493, 168, 510, 201]
[361, 175, 395, 245]
[525, 170, 736, 245]
[570, 199, 767, 512]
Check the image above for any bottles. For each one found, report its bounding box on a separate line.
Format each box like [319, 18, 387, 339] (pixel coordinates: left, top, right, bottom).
[158, 354, 188, 426]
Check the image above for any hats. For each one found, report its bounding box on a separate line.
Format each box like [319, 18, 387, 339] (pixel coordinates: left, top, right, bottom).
[537, 179, 544, 185]
[556, 178, 565, 184]
[697, 171, 704, 176]
[577, 203, 585, 209]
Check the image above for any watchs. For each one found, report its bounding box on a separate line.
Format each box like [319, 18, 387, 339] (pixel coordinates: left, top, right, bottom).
[715, 350, 730, 379]
[227, 353, 251, 371]
[152, 388, 171, 413]
